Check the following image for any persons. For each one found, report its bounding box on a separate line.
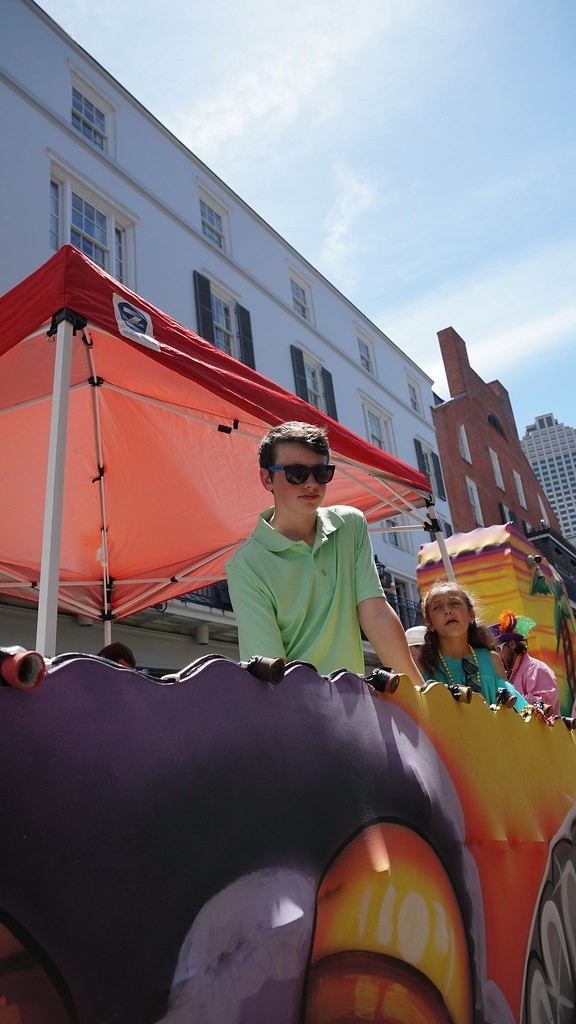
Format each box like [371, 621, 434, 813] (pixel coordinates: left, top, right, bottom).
[227, 420, 426, 690]
[487, 623, 560, 721]
[405, 626, 430, 673]
[97, 642, 136, 668]
[420, 581, 529, 714]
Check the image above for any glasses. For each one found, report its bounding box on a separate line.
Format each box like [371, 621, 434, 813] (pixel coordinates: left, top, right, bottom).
[267, 463, 335, 484]
[494, 641, 508, 654]
[462, 657, 482, 695]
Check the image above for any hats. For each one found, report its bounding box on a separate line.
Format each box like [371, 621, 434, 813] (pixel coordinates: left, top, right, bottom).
[405, 626, 427, 646]
[487, 611, 536, 645]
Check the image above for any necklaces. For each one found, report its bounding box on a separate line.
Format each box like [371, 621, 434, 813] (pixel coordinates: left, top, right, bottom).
[507, 654, 520, 681]
[438, 645, 480, 686]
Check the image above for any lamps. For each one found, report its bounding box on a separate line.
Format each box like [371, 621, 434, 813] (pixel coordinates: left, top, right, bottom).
[0, 645, 46, 692]
[533, 700, 555, 717]
[561, 716, 576, 729]
[246, 655, 285, 685]
[77, 614, 94, 628]
[446, 684, 472, 703]
[496, 686, 517, 708]
[371, 667, 400, 693]
[195, 623, 209, 646]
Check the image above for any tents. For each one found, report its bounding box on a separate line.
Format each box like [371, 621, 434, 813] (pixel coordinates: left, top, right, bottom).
[0, 243, 455, 667]
